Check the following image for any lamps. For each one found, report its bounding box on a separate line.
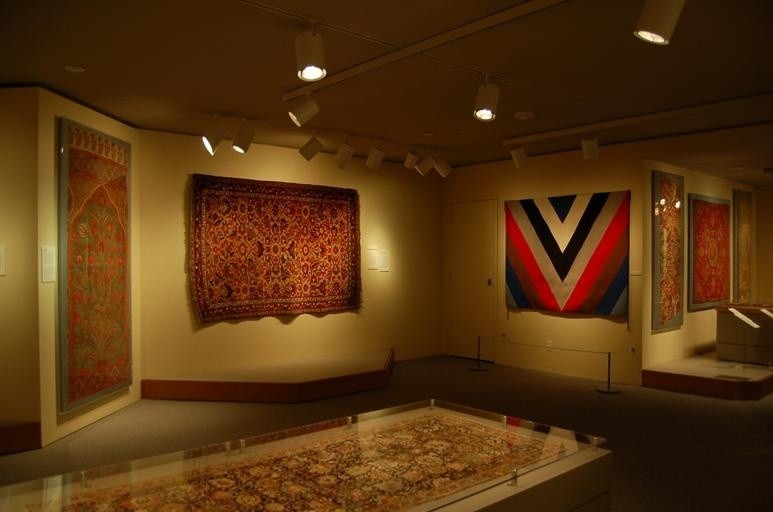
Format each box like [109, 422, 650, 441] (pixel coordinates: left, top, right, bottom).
[288, 100, 319, 127]
[509, 145, 527, 169]
[362, 146, 385, 171]
[633, 1, 684, 45]
[402, 151, 453, 179]
[294, 21, 327, 83]
[203, 113, 232, 157]
[332, 141, 355, 171]
[472, 74, 501, 123]
[298, 134, 324, 161]
[581, 138, 600, 162]
[232, 117, 260, 154]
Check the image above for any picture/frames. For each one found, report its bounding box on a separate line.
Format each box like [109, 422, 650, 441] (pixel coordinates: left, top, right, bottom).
[649, 168, 687, 335]
[685, 190, 731, 316]
[55, 112, 133, 423]
